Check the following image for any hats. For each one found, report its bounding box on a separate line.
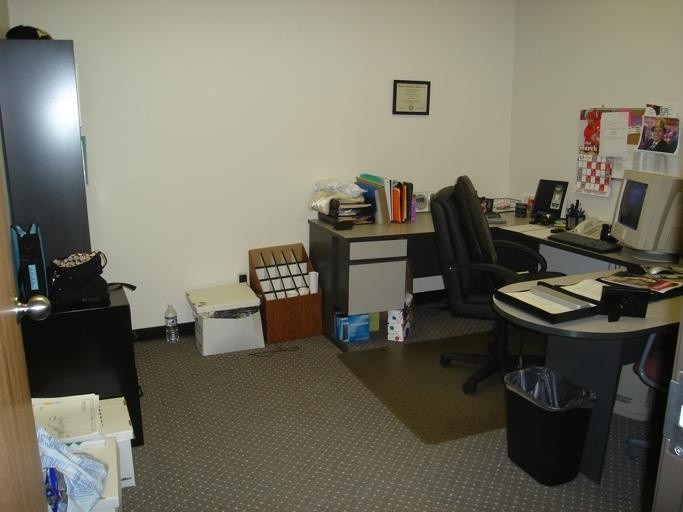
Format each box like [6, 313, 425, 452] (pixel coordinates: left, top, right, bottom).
[650, 117, 669, 134]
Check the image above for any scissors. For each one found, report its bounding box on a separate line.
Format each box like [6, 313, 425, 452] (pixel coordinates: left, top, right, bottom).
[568, 206, 577, 215]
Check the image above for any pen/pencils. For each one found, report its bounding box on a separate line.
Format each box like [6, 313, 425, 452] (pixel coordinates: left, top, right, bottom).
[566, 199, 585, 217]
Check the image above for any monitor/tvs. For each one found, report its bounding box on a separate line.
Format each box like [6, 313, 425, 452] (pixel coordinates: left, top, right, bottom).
[610, 170, 683, 262]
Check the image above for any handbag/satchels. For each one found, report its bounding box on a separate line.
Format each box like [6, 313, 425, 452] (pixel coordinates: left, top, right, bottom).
[45, 249, 112, 311]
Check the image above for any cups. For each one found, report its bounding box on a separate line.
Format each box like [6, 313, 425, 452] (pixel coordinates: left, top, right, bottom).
[515, 203, 528, 217]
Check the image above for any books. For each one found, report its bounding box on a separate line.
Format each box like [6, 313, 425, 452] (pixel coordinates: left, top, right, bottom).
[318, 173, 416, 227]
[31, 392, 106, 449]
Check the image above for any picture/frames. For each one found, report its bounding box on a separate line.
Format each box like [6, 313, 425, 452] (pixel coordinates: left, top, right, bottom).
[392, 79, 431, 116]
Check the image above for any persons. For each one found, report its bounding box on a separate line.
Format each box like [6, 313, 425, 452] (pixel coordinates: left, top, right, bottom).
[640, 127, 673, 153]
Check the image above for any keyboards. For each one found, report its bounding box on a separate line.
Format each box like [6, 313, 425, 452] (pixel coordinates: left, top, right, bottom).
[547, 231, 621, 254]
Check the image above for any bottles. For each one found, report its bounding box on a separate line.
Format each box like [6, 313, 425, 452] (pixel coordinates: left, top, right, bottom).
[165, 302, 180, 345]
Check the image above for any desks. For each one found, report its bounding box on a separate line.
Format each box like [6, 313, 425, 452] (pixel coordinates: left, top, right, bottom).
[308, 205, 683, 485]
[62, 439, 123, 512]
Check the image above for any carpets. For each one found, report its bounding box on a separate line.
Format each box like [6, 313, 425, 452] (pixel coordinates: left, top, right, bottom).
[338, 332, 547, 445]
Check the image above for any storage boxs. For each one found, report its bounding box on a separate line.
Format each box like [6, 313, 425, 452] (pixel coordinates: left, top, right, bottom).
[29, 396, 137, 489]
[187, 282, 265, 356]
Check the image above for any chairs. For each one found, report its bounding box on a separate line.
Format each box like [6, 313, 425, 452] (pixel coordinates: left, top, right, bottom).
[430, 175, 567, 396]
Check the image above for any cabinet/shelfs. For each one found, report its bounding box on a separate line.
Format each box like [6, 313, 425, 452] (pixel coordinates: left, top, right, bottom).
[0, 39, 92, 264]
[21, 284, 144, 446]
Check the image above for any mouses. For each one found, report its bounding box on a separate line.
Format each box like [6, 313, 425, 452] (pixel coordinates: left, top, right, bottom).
[649, 266, 675, 275]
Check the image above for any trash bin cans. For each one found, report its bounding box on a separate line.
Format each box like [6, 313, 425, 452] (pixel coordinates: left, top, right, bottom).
[503, 366, 600, 486]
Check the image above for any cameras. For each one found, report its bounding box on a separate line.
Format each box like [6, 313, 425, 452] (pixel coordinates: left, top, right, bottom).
[534, 211, 557, 225]
